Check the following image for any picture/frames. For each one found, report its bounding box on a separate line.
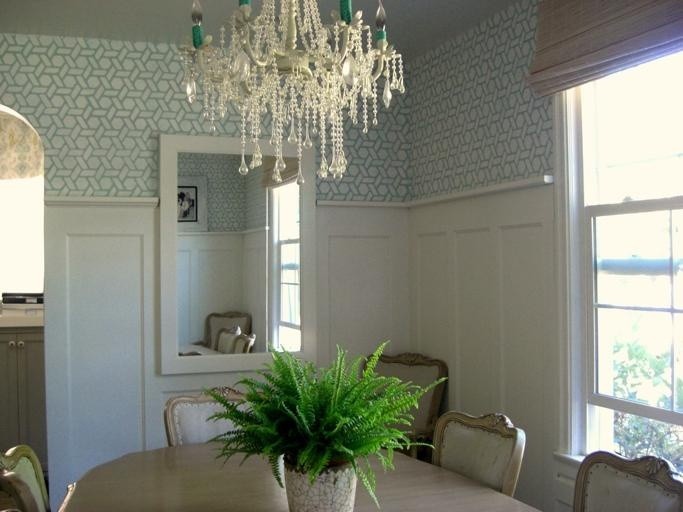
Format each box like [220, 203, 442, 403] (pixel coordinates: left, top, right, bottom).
[176, 185, 197, 222]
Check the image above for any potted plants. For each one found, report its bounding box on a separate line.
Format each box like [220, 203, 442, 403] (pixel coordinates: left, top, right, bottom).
[206, 341, 448, 511]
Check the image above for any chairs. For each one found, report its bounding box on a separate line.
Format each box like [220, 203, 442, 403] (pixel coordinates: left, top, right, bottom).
[232, 333, 255, 354]
[163, 386, 266, 448]
[1, 445, 50, 512]
[430, 411, 527, 499]
[572, 451, 642, 511]
[193, 310, 250, 347]
[362, 352, 448, 457]
[180, 351, 204, 357]
[215, 328, 241, 354]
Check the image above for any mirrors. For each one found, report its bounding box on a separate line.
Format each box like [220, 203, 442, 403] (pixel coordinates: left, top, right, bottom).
[158, 134, 316, 374]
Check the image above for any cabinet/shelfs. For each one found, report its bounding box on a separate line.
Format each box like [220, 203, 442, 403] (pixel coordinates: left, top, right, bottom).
[0, 328, 48, 484]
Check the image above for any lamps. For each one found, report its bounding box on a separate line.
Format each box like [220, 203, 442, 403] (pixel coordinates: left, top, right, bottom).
[177, 1, 405, 185]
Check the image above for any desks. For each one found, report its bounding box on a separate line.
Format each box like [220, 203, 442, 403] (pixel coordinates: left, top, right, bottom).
[59, 437, 538, 512]
